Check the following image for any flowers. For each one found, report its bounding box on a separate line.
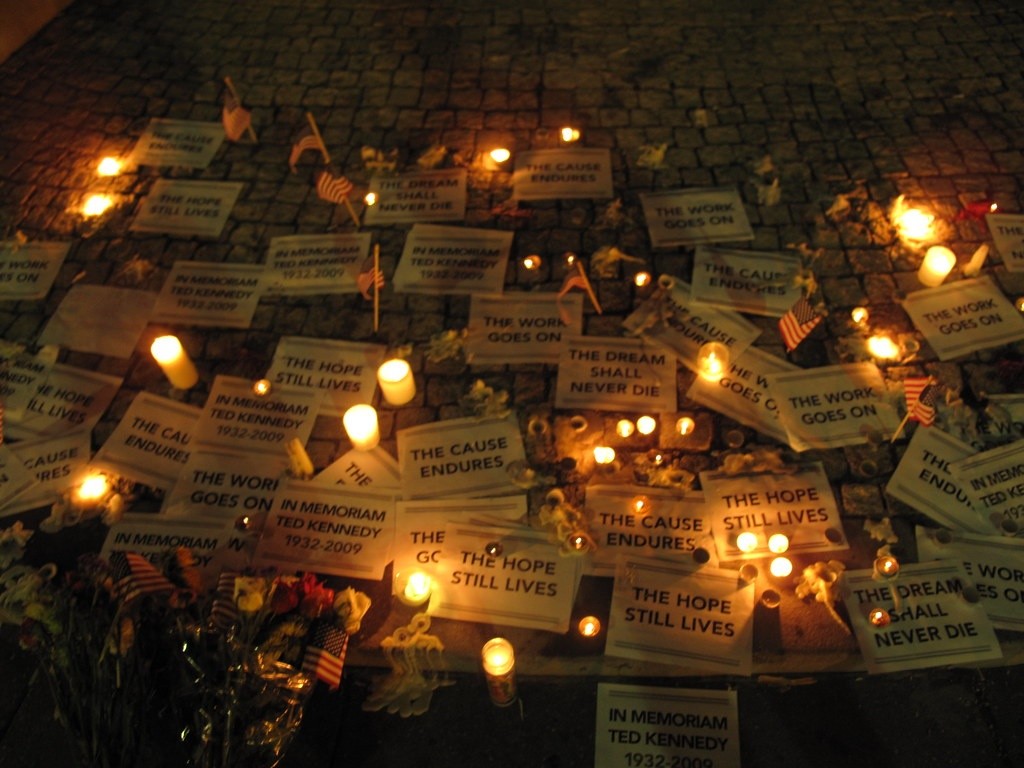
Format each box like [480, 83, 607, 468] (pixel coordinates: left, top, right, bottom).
[0, 523, 372, 767]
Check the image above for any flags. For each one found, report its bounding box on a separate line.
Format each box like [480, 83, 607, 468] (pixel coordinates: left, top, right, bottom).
[904, 378, 937, 426]
[317, 160, 353, 204]
[779, 296, 824, 351]
[108, 544, 348, 691]
[357, 244, 384, 301]
[289, 113, 324, 174]
[557, 261, 588, 300]
[224, 86, 250, 141]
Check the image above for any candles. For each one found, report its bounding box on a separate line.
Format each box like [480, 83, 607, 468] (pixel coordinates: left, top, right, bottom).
[376, 358, 417, 405]
[342, 403, 380, 452]
[578, 615, 601, 637]
[152, 335, 198, 390]
[918, 246, 957, 288]
[483, 637, 517, 708]
